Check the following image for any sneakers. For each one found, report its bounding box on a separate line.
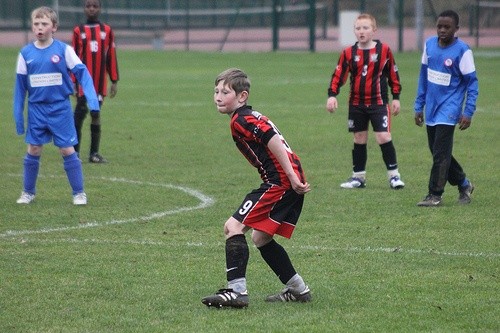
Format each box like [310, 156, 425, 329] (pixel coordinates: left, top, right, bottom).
[201, 288, 249, 309]
[266, 284, 311, 303]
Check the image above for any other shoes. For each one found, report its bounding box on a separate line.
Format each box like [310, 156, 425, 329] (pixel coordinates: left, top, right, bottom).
[416, 193, 444, 206]
[390, 175, 405, 189]
[457, 179, 474, 204]
[340, 177, 366, 189]
[89, 152, 109, 163]
[73, 192, 87, 205]
[16, 191, 36, 204]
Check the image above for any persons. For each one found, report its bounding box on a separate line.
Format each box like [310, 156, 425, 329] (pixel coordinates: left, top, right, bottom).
[200, 68, 312, 309]
[13, 7, 100, 205]
[70, 0, 120, 163]
[326, 13, 405, 189]
[414, 10, 479, 206]
